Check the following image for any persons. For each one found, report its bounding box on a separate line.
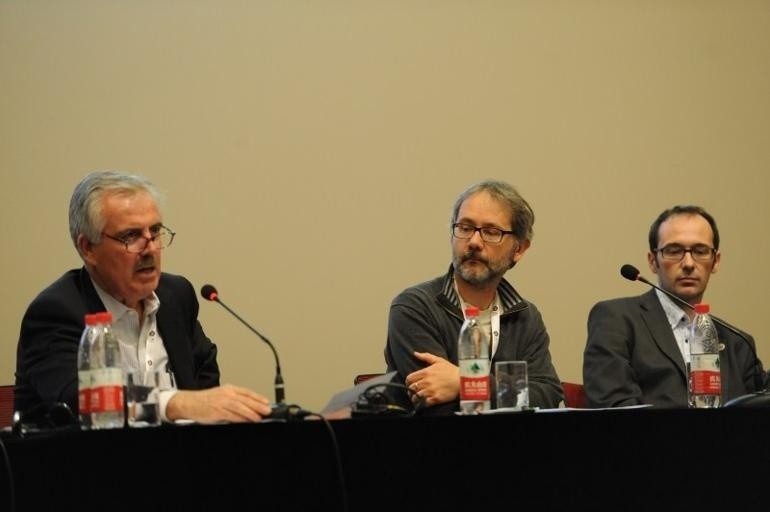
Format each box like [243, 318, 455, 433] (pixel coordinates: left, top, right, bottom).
[584, 205, 769, 408]
[11, 168, 273, 430]
[379, 182, 569, 416]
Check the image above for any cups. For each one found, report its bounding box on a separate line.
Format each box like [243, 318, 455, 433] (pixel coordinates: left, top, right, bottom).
[126, 371, 161, 428]
[495, 361, 529, 409]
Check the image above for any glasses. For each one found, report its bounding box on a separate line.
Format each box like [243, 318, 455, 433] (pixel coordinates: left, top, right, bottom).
[655, 243, 718, 266]
[100, 222, 176, 253]
[451, 219, 514, 245]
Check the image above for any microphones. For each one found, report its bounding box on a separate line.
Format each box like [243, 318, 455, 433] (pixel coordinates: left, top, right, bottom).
[620, 263, 770, 410]
[199, 284, 309, 425]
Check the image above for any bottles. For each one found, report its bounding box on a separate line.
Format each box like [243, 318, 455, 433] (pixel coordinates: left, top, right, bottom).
[685, 303, 723, 408]
[457, 307, 492, 414]
[75, 312, 125, 431]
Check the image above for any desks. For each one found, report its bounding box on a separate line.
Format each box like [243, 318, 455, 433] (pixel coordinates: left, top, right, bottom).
[1, 406, 770, 511]
